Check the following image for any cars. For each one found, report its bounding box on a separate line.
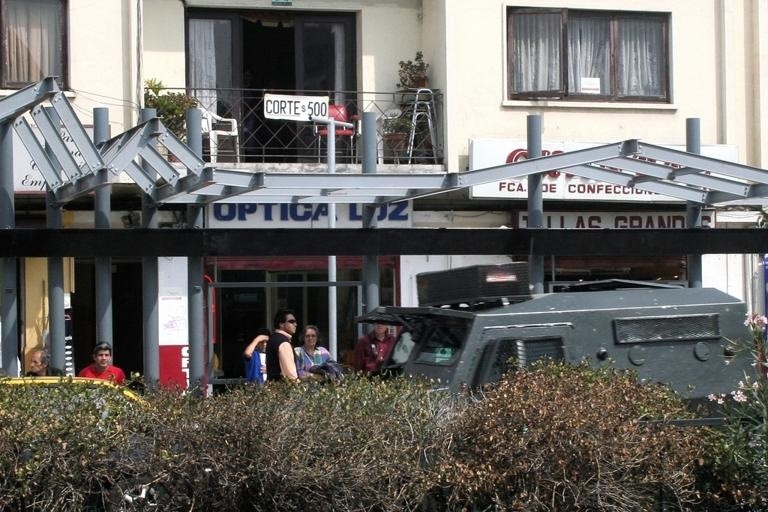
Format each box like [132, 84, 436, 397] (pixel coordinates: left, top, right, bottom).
[0, 375, 156, 437]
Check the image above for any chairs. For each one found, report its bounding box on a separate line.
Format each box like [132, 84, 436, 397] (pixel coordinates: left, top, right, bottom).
[312, 104, 362, 164]
[196, 106, 240, 163]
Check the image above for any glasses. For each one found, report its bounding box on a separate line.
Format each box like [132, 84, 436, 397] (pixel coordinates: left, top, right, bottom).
[287, 320, 296, 324]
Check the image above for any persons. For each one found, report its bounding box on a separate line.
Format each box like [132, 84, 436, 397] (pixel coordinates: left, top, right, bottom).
[75, 339, 126, 384]
[263, 307, 298, 381]
[289, 321, 335, 381]
[22, 349, 64, 375]
[241, 327, 271, 384]
[352, 320, 396, 380]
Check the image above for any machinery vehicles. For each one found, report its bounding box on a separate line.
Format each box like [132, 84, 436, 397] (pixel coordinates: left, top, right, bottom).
[352, 261, 760, 433]
[222, 274, 343, 378]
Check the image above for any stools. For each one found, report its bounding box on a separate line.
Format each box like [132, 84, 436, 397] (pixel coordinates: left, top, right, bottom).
[398, 89, 442, 164]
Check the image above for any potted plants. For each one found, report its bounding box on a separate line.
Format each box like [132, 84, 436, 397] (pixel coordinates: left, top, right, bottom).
[143, 79, 199, 162]
[381, 117, 417, 154]
[396, 50, 432, 89]
